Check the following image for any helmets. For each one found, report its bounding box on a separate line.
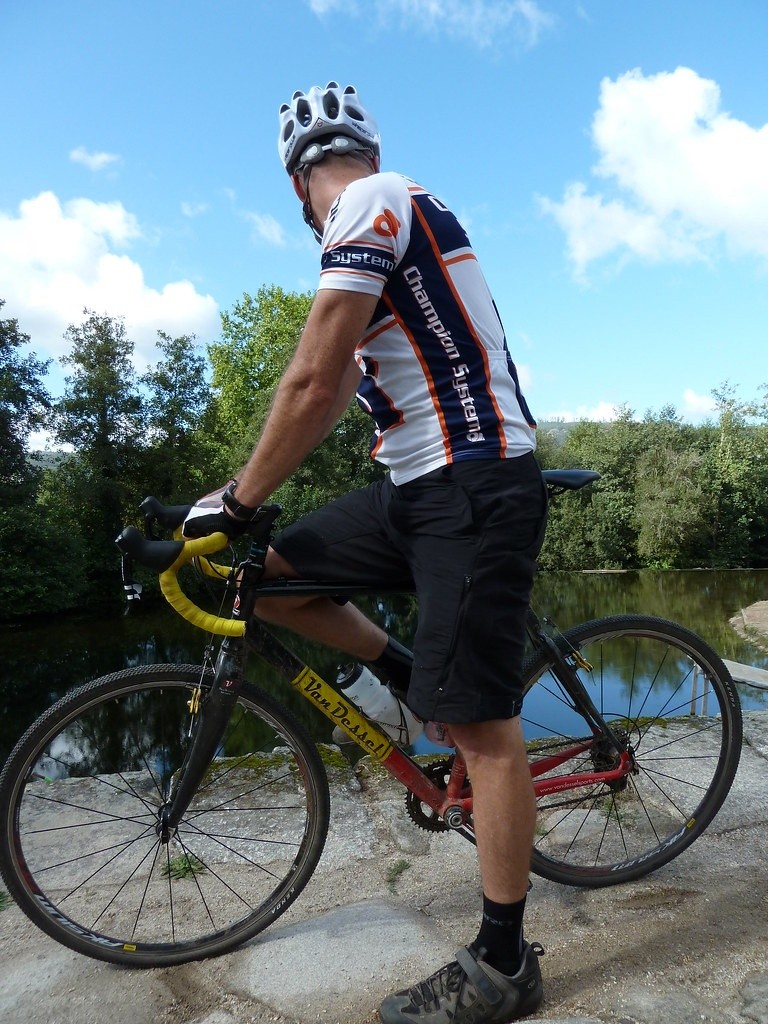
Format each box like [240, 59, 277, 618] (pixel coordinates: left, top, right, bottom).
[278, 80, 380, 172]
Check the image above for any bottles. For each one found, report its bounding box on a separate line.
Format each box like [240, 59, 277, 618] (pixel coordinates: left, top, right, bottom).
[337, 660, 422, 745]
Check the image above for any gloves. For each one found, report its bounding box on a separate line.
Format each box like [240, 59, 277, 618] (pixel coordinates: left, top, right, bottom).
[181, 506, 249, 541]
[193, 479, 238, 508]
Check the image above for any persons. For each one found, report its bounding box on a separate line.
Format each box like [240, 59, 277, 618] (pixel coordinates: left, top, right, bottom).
[175, 81, 549, 1024]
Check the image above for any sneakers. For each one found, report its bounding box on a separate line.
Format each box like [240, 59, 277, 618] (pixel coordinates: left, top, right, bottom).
[378, 941, 544, 1023]
[332, 711, 374, 745]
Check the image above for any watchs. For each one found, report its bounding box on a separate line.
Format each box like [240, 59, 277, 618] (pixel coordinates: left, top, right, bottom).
[221, 483, 261, 521]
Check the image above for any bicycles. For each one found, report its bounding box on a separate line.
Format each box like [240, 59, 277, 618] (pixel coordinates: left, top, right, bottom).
[1, 468, 743, 969]
